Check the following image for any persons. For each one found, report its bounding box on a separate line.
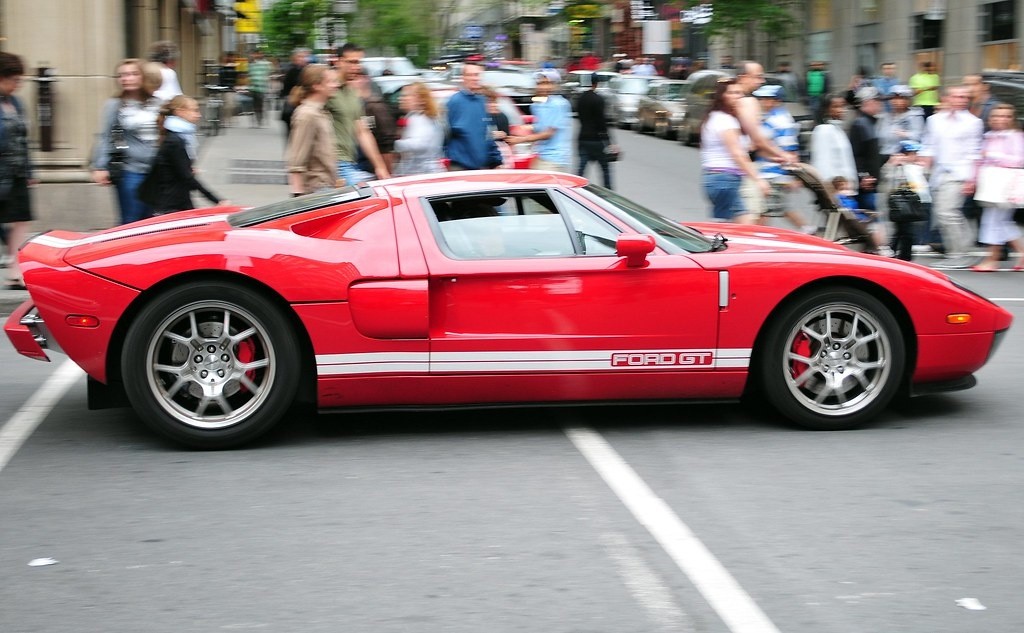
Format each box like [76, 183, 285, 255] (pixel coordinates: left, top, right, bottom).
[0, 51, 35, 290]
[504, 68, 573, 214]
[700, 60, 1024, 273]
[219, 51, 274, 129]
[139, 95, 231, 219]
[612, 53, 734, 80]
[91, 41, 197, 226]
[396, 82, 449, 173]
[281, 43, 619, 215]
[834, 177, 894, 257]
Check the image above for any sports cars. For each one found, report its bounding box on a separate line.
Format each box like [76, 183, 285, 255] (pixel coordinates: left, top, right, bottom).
[2, 169, 1015, 452]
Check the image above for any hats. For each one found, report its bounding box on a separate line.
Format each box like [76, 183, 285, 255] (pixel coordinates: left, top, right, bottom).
[752, 84, 788, 100]
[888, 84, 913, 97]
[852, 85, 879, 103]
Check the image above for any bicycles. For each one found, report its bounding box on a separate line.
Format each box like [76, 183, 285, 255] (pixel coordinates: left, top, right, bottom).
[196, 72, 228, 138]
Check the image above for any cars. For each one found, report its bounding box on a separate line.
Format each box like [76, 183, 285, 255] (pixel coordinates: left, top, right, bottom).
[357, 54, 817, 159]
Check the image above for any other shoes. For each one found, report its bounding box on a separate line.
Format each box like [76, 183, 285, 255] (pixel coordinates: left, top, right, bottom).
[4, 275, 28, 290]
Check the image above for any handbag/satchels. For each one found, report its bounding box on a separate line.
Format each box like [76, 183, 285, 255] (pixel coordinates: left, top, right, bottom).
[86, 98, 128, 183]
[887, 164, 932, 222]
